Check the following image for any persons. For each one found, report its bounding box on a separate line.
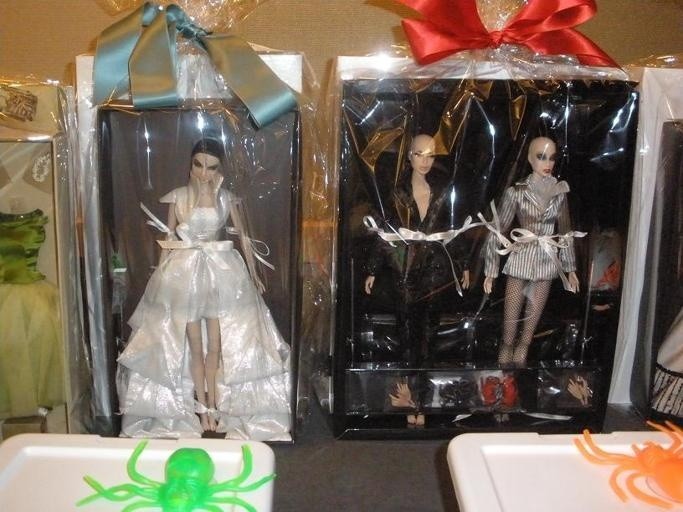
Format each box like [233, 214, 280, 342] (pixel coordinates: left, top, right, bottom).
[482, 137, 581, 424]
[363, 134, 471, 425]
[113, 128, 293, 443]
